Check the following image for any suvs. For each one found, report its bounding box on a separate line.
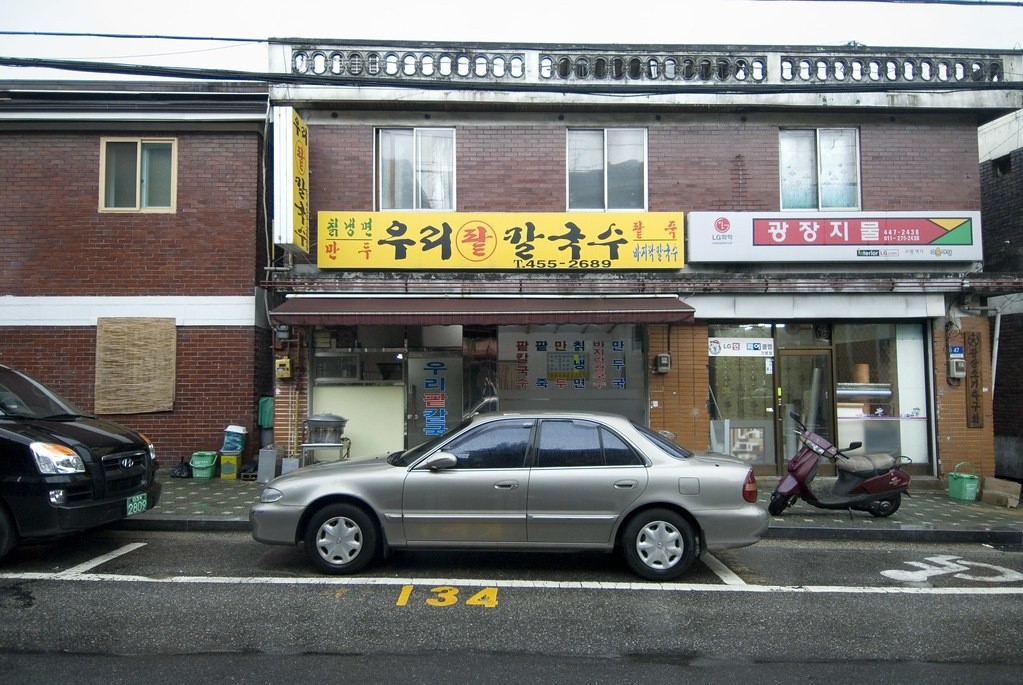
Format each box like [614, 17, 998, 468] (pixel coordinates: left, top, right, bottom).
[0, 364, 162, 565]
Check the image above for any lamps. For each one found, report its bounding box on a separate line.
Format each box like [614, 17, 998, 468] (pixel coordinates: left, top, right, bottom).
[946, 319, 960, 339]
[961, 261, 985, 283]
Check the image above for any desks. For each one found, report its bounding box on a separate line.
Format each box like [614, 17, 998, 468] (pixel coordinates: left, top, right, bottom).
[300, 438, 351, 467]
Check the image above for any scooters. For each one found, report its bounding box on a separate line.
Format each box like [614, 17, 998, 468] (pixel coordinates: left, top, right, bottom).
[767, 411, 912, 518]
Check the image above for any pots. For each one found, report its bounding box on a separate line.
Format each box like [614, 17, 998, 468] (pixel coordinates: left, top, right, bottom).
[306, 413, 348, 428]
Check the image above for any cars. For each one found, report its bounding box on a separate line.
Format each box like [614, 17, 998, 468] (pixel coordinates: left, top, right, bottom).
[250, 411, 770, 583]
